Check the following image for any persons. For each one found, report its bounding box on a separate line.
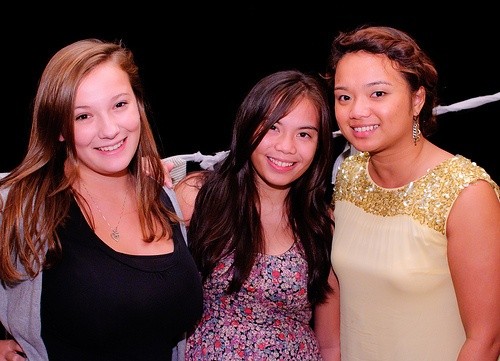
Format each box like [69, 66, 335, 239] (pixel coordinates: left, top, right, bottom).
[320, 24, 500, 360]
[0, 37, 232, 360]
[171, 70, 344, 361]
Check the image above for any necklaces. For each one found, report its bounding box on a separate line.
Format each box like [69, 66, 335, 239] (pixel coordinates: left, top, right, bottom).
[77, 172, 134, 242]
